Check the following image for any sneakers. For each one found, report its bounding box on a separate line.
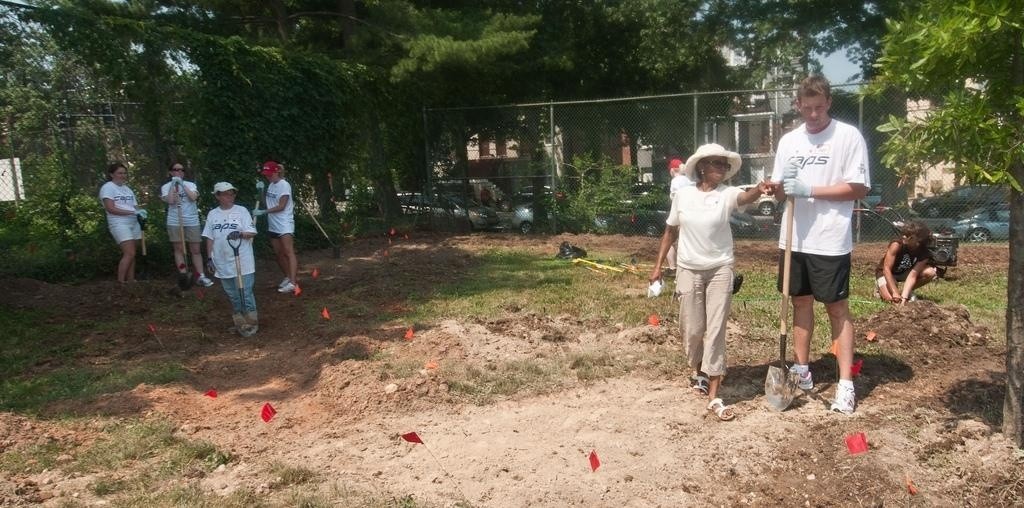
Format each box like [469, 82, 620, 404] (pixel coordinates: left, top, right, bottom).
[277, 277, 300, 294]
[784, 364, 816, 393]
[831, 378, 859, 413]
[234, 316, 263, 338]
[193, 272, 215, 288]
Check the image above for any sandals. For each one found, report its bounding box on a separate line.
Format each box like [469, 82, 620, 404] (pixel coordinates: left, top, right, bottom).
[691, 376, 709, 397]
[708, 396, 735, 421]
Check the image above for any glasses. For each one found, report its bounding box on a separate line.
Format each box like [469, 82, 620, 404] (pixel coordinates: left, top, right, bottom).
[700, 158, 734, 171]
[170, 167, 186, 173]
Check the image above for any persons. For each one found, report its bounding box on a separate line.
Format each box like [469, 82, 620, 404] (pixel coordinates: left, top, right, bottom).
[770, 73, 874, 417]
[160, 162, 215, 288]
[875, 218, 936, 307]
[201, 181, 259, 337]
[651, 142, 780, 424]
[662, 157, 684, 276]
[252, 160, 297, 292]
[99, 162, 146, 283]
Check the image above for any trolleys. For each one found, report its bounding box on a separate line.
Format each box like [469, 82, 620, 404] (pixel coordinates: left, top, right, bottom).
[845, 203, 961, 278]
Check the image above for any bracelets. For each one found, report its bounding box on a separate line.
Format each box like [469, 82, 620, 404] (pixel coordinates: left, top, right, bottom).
[901, 297, 909, 300]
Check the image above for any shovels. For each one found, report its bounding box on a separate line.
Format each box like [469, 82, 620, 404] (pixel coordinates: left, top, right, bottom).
[136, 215, 150, 282]
[299, 198, 341, 259]
[226, 236, 259, 338]
[764, 196, 802, 411]
[175, 181, 194, 291]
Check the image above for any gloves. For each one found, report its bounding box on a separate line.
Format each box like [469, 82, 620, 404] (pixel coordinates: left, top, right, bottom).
[779, 157, 820, 201]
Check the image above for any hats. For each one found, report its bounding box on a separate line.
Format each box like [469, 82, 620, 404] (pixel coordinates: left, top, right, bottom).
[261, 162, 286, 177]
[212, 181, 239, 195]
[682, 141, 744, 185]
